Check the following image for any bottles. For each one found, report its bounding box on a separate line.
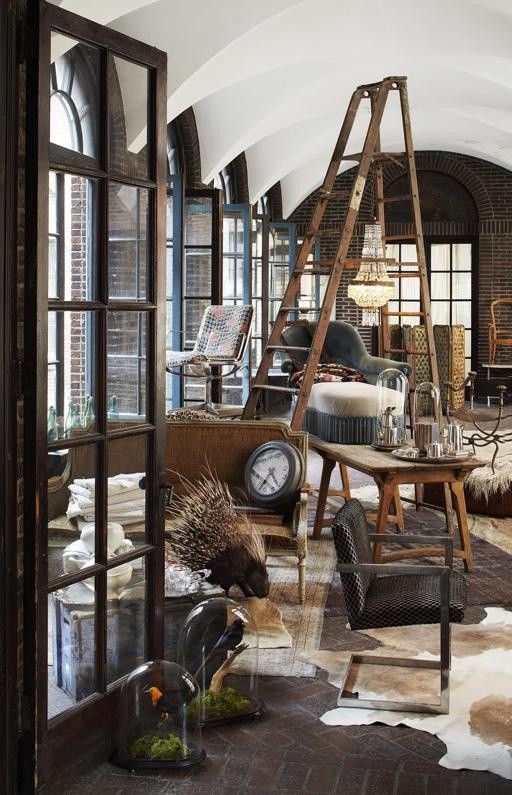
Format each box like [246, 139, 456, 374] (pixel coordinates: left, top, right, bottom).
[48, 395, 119, 441]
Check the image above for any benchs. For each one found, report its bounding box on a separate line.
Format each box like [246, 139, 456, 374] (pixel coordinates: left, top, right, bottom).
[48, 417, 310, 608]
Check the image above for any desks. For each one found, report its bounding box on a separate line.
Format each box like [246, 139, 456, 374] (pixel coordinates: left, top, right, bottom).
[308, 435, 485, 574]
[480, 361, 511, 409]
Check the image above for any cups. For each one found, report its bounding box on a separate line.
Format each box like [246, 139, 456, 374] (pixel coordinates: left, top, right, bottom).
[170, 565, 192, 593]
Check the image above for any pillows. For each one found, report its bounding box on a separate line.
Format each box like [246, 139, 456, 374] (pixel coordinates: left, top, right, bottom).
[421, 485, 511, 556]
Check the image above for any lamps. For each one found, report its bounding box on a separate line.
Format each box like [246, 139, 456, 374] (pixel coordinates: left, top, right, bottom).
[344, 167, 395, 311]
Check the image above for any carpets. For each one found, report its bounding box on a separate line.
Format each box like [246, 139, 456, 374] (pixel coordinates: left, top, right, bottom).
[226, 505, 337, 678]
[296, 606, 512, 781]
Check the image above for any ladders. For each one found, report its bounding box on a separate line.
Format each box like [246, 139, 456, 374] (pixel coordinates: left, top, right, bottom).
[241, 77, 444, 432]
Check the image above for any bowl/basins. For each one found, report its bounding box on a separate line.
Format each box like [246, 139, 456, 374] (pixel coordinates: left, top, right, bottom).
[81, 556, 134, 590]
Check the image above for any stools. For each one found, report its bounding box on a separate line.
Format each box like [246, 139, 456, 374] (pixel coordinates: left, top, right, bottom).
[292, 379, 405, 445]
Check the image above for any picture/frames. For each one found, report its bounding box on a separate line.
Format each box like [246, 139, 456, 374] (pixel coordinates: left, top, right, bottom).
[268, 319, 330, 370]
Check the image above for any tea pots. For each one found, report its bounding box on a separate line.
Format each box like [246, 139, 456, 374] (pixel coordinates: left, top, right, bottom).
[426, 442, 449, 459]
[448, 424, 464, 452]
[383, 426, 404, 446]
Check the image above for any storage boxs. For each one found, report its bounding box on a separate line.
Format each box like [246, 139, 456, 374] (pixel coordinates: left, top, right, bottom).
[50, 567, 226, 704]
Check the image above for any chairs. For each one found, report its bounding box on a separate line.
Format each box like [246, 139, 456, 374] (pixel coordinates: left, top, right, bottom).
[165, 304, 254, 418]
[277, 318, 413, 390]
[485, 296, 512, 365]
[330, 497, 467, 715]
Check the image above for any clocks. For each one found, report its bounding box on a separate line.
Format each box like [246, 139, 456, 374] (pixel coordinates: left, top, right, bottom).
[241, 438, 307, 511]
